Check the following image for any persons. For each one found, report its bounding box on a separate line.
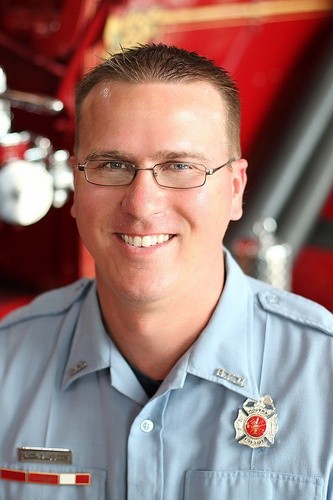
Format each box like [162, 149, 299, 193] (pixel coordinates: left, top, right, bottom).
[0, 41, 333, 500]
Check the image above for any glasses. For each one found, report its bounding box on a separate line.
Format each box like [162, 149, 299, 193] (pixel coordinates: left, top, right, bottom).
[75, 156, 237, 189]
